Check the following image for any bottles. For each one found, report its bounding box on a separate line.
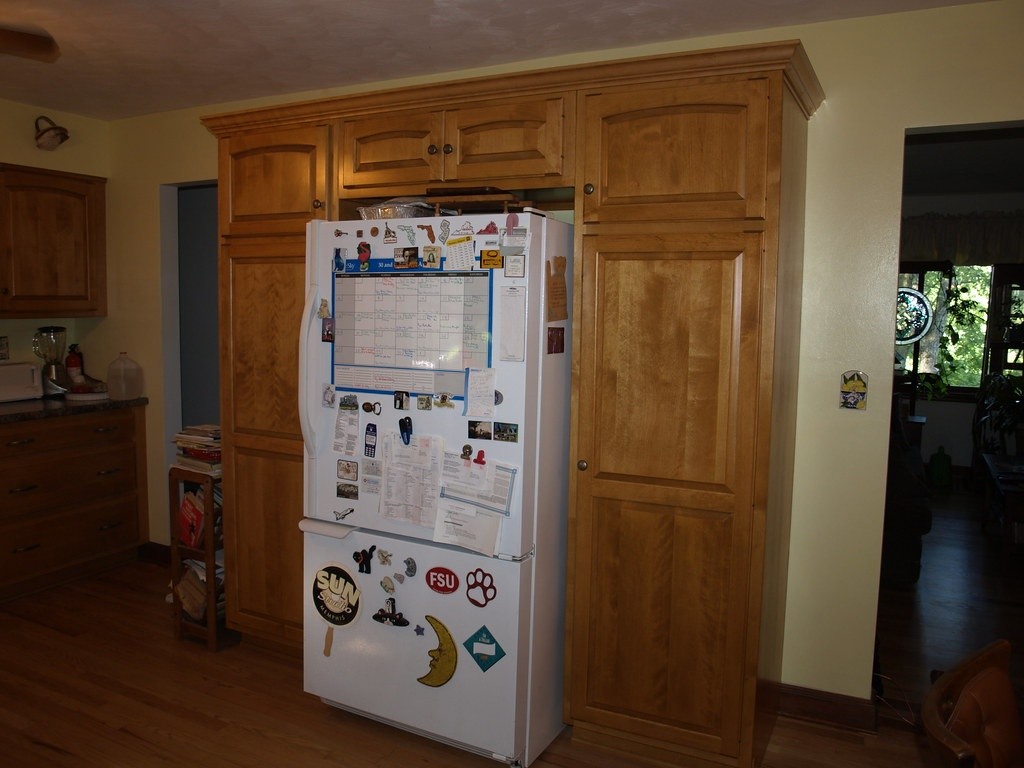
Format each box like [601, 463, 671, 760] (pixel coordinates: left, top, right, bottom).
[107, 352, 143, 400]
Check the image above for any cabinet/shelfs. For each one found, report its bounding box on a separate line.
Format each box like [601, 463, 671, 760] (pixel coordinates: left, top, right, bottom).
[168, 465, 225, 652]
[0, 160, 108, 319]
[893, 261, 952, 452]
[0, 395, 149, 605]
[970, 342, 1024, 469]
[199, 38, 826, 768]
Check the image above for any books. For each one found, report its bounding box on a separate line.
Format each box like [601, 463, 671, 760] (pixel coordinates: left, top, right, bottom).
[175, 559, 223, 625]
[169, 424, 221, 476]
[176, 482, 223, 548]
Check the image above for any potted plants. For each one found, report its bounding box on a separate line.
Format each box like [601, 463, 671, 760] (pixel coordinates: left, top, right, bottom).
[986, 310, 1011, 343]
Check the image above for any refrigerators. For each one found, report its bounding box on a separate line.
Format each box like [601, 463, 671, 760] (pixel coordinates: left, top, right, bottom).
[296, 207, 575, 768]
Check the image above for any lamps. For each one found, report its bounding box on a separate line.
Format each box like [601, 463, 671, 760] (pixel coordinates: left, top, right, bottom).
[34, 116, 71, 151]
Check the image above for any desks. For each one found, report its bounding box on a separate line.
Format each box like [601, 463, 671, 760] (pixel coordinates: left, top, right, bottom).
[980, 453, 1024, 578]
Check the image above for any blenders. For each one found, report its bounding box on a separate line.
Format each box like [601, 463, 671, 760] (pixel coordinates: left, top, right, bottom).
[31, 325, 67, 399]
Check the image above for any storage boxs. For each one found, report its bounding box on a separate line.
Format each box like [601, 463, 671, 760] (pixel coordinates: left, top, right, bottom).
[183, 448, 221, 464]
